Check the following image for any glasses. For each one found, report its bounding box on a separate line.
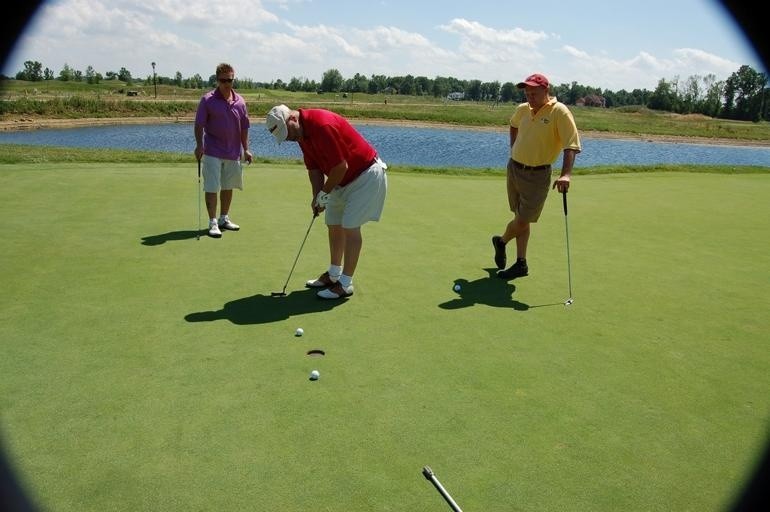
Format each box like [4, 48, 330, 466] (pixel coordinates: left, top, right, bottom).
[219, 78, 233, 83]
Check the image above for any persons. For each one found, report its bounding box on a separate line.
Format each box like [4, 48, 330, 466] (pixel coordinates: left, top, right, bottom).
[193, 62, 254, 238]
[491, 73, 582, 280]
[266, 104, 389, 300]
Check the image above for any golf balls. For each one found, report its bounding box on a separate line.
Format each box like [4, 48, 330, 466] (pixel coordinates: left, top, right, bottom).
[312, 371, 319, 379]
[454, 285, 460, 291]
[296, 327, 303, 334]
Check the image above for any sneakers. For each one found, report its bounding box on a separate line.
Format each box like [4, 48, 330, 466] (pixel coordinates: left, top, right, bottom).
[317, 281, 354, 300]
[492, 235, 506, 269]
[208, 223, 222, 238]
[218, 219, 240, 230]
[498, 263, 528, 280]
[306, 271, 340, 288]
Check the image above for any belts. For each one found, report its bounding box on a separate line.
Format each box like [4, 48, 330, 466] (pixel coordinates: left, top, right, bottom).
[511, 159, 551, 171]
[360, 154, 377, 173]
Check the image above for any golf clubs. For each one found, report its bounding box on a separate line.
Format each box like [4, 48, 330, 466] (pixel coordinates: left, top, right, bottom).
[271, 207, 318, 297]
[198, 157, 200, 240]
[563, 186, 573, 305]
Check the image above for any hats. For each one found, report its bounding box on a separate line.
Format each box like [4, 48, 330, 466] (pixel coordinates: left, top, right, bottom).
[516, 74, 549, 90]
[265, 104, 289, 144]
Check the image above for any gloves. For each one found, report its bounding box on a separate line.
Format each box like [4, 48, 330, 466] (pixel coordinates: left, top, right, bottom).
[315, 191, 328, 208]
